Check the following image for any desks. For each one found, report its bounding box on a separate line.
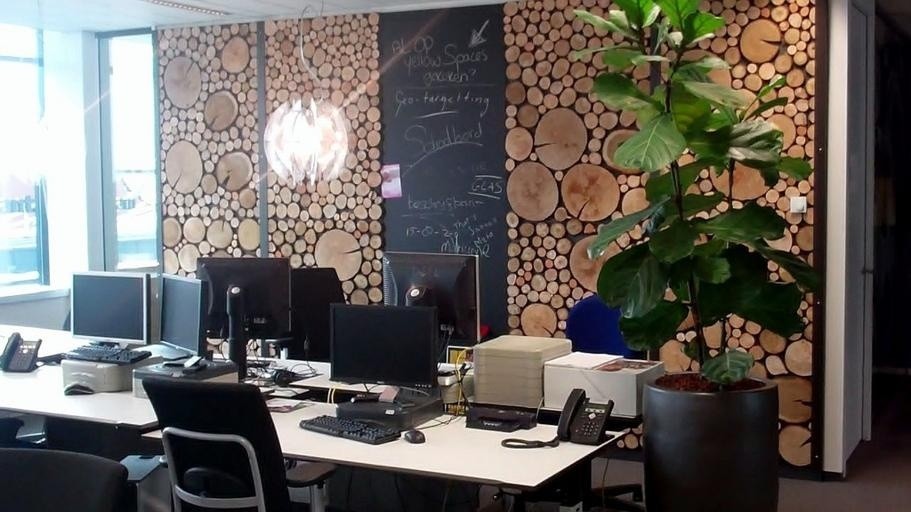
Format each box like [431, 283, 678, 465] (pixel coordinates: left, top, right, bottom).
[0, 322, 632, 512]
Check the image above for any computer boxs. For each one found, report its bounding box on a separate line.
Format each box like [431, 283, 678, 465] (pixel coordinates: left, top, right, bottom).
[336, 394, 444, 431]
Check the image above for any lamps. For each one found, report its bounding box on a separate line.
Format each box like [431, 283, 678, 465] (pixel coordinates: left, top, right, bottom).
[264, 0, 351, 189]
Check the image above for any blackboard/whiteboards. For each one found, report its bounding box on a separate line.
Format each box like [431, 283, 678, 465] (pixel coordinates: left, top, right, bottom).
[378, 4, 512, 345]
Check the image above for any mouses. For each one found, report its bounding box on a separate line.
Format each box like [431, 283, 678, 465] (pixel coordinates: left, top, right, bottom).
[405, 430, 425, 444]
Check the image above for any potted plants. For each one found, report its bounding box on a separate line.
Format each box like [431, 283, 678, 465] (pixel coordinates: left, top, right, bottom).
[565, 1, 830, 511]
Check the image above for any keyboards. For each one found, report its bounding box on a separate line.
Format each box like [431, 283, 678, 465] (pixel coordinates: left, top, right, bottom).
[66, 345, 152, 366]
[299, 414, 401, 445]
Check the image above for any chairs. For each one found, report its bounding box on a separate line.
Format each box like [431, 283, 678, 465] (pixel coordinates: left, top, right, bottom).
[264, 267, 348, 363]
[138, 373, 339, 512]
[564, 294, 650, 511]
[0, 444, 131, 512]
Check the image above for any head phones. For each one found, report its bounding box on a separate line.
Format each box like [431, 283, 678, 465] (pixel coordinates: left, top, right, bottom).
[272, 368, 301, 387]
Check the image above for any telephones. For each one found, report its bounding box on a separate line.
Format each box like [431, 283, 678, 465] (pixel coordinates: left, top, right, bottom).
[557, 389, 614, 446]
[0, 333, 42, 372]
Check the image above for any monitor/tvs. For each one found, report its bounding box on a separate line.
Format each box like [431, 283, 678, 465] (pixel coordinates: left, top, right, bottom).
[329, 302, 440, 408]
[159, 273, 209, 374]
[70, 270, 150, 351]
[196, 257, 292, 400]
[382, 251, 480, 376]
